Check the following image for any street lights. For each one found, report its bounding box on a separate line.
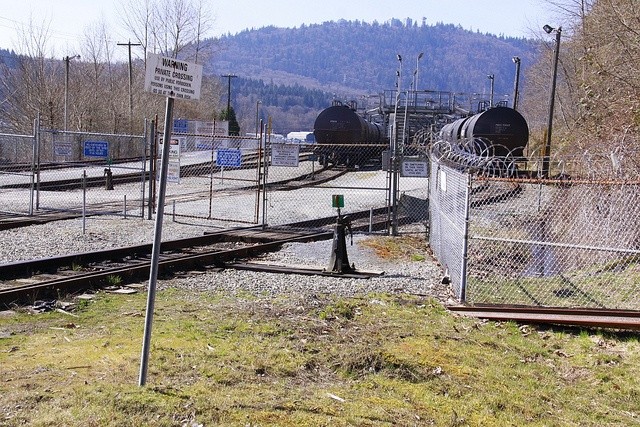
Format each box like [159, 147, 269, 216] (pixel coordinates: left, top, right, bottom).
[542, 24, 562, 182]
[410, 52, 423, 106]
[511, 56, 522, 109]
[393, 54, 402, 108]
[487, 74, 495, 107]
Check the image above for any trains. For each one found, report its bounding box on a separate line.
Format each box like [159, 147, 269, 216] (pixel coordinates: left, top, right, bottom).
[440, 103, 529, 163]
[314, 100, 388, 169]
